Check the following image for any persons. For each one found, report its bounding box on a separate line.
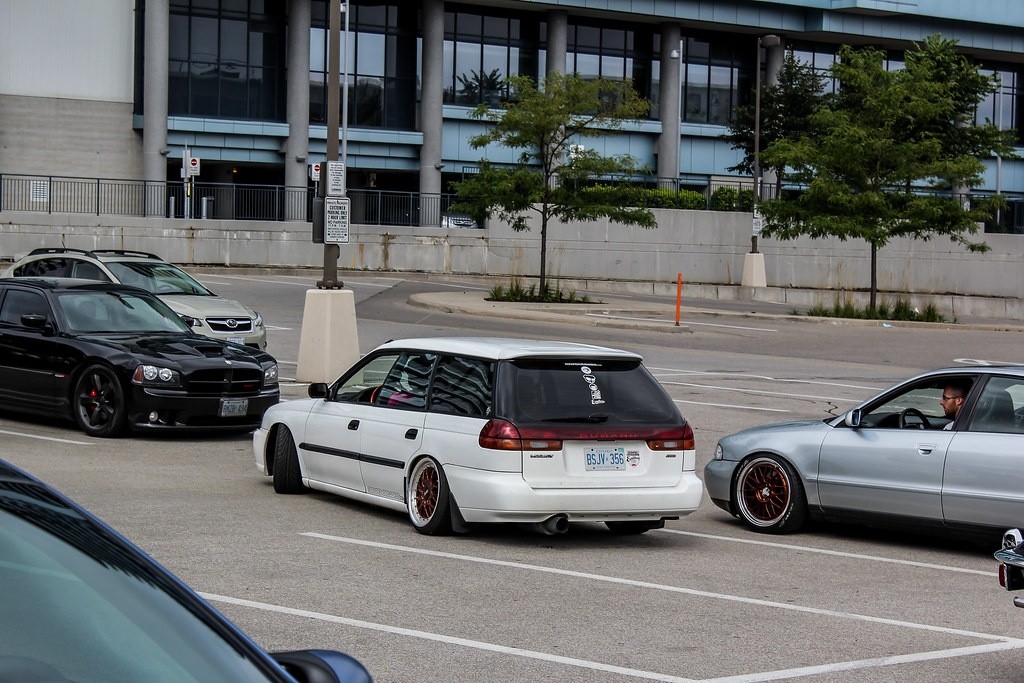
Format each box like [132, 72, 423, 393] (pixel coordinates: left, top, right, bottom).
[388, 358, 431, 409]
[76, 301, 144, 330]
[941, 378, 973, 430]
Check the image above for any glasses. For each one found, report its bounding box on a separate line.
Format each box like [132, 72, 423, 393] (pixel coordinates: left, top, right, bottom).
[942, 394, 965, 402]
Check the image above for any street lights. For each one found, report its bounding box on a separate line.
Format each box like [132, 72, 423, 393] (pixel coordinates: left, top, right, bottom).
[670, 39, 683, 193]
[742, 34, 781, 287]
[985, 72, 1003, 227]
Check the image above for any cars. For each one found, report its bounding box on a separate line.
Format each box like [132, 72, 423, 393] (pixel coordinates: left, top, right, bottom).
[252, 337, 703, 537]
[440, 212, 479, 228]
[703, 365, 1024, 535]
[0, 459, 375, 683]
[0, 276, 281, 438]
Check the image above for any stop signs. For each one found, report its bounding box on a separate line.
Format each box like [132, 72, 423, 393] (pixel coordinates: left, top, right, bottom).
[191, 159, 197, 166]
[314, 165, 321, 172]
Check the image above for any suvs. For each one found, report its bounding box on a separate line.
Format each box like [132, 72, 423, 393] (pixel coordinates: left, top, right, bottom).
[0, 246, 270, 352]
